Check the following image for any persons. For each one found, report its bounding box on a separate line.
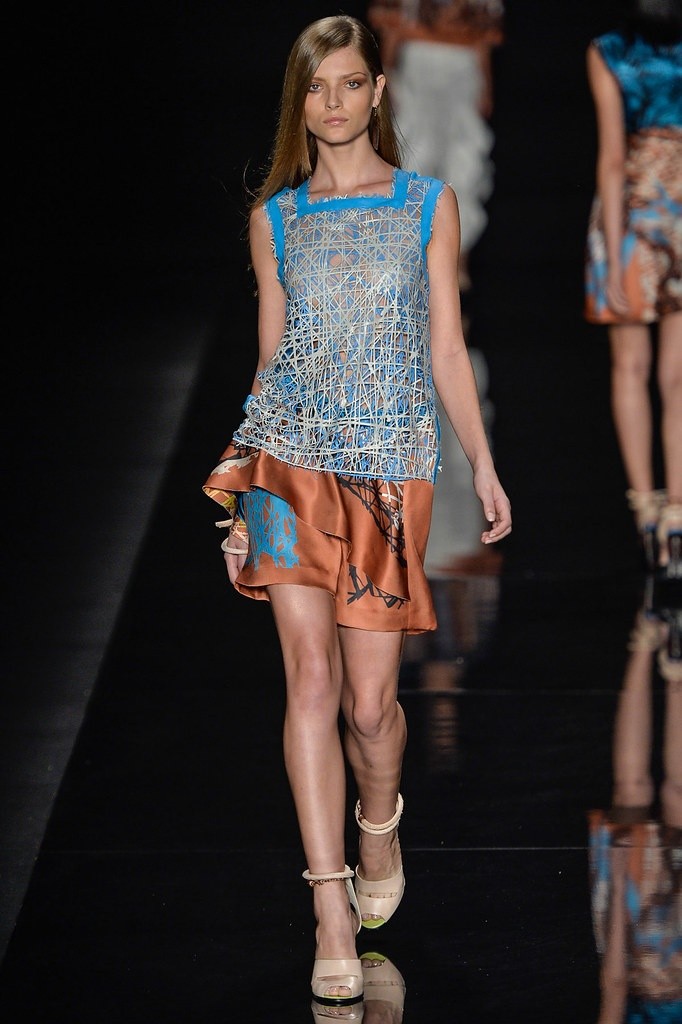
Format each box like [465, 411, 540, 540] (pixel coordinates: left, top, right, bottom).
[584, 0, 682, 571]
[368, 0, 505, 290]
[201, 16, 512, 1004]
[588, 611, 682, 1024]
[408, 344, 504, 777]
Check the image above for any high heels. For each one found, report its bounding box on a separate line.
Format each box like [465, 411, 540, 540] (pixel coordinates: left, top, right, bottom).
[624, 488, 661, 568]
[654, 500, 682, 568]
[352, 792, 406, 929]
[355, 951, 406, 1023]
[302, 864, 365, 1006]
[625, 574, 682, 685]
[308, 992, 365, 1024]
